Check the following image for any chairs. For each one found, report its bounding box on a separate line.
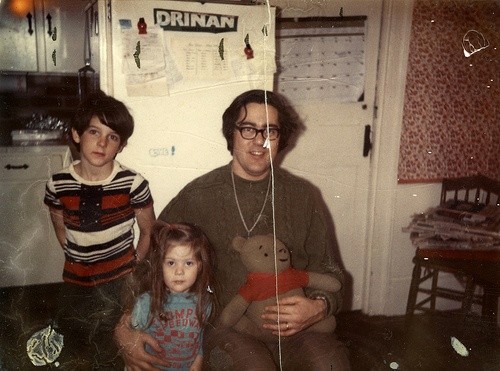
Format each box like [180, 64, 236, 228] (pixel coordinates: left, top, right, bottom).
[404, 176, 500, 328]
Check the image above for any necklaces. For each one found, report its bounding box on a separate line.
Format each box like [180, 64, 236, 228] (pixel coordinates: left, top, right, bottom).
[231, 160, 272, 238]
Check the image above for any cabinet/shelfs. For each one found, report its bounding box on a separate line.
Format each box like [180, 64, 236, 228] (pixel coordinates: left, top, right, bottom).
[0, 145, 75, 289]
[1, 0, 86, 73]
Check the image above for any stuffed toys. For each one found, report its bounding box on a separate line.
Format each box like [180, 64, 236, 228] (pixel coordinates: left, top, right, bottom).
[220, 232, 341, 343]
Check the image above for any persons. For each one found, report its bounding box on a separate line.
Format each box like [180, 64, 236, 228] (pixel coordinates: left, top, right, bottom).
[115, 88, 354, 371]
[126, 219, 214, 370]
[48, 90, 155, 370]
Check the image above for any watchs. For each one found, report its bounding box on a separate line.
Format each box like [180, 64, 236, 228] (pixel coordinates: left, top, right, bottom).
[316, 295, 330, 319]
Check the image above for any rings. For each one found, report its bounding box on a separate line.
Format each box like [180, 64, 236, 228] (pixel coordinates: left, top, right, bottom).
[285, 322, 288, 330]
